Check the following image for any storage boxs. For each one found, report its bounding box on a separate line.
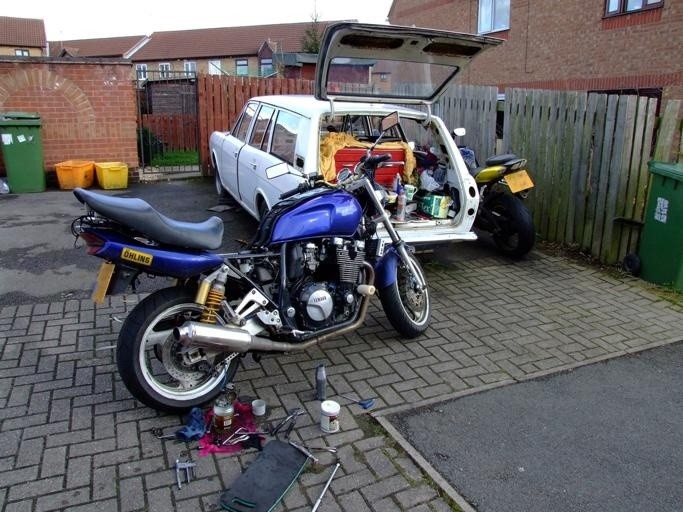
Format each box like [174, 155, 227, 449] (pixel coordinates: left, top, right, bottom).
[95, 162, 130, 191]
[55, 160, 96, 189]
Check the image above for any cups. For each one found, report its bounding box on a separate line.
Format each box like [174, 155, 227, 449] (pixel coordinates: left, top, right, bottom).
[403, 184, 417, 200]
[253, 401, 266, 416]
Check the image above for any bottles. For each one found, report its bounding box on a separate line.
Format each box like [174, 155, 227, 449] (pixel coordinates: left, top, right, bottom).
[212, 390, 235, 433]
[315, 364, 327, 400]
[397, 192, 407, 222]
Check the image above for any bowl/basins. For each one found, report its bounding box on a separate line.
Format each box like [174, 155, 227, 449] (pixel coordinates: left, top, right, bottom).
[384, 191, 397, 204]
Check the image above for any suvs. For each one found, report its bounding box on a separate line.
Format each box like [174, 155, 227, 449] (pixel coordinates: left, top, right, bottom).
[207, 24, 506, 261]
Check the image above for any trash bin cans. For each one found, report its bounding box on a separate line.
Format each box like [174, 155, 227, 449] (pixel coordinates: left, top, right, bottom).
[0, 112, 46, 192]
[624, 160, 683, 292]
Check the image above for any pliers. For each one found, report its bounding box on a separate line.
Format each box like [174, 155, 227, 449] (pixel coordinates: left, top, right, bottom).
[271, 409, 305, 438]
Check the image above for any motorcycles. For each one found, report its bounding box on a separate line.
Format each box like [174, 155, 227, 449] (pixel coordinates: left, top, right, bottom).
[451, 127, 535, 262]
[70, 112, 430, 416]
